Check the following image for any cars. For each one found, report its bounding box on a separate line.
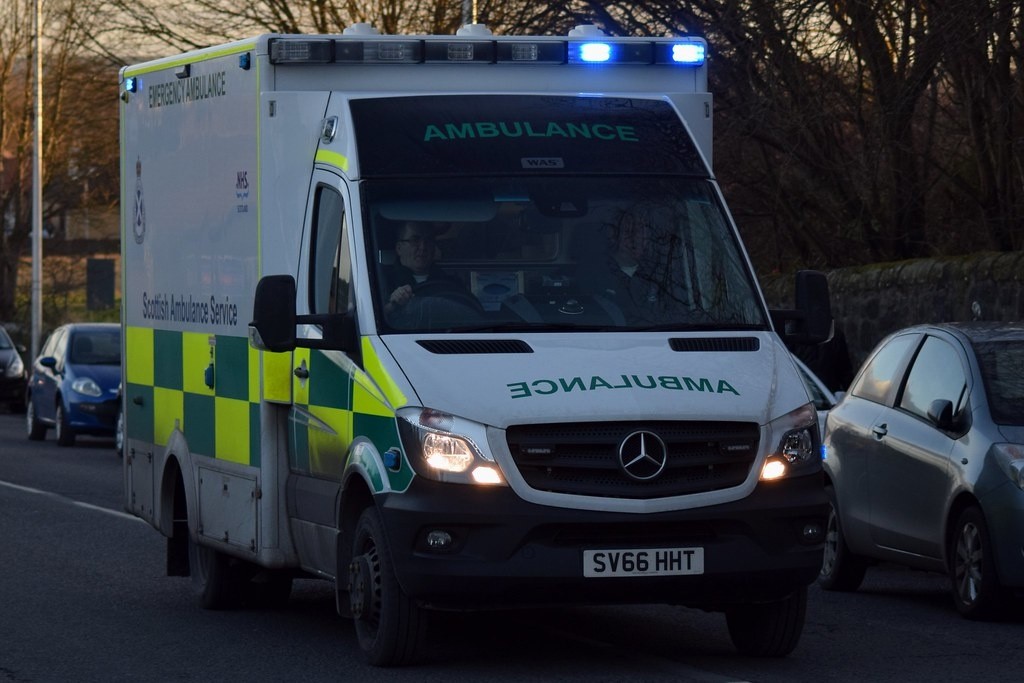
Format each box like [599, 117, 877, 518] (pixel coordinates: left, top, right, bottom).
[821, 318, 1024, 627]
[0, 324, 29, 414]
[26, 322, 122, 447]
[790, 353, 849, 444]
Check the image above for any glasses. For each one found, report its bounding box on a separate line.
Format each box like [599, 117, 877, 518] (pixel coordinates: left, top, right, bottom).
[402, 236, 434, 248]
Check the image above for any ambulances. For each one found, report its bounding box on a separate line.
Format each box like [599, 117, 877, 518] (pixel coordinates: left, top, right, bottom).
[117, 23, 836, 669]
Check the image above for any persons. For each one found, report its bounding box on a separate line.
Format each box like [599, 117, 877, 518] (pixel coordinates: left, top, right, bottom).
[585, 214, 665, 323]
[376, 221, 464, 329]
